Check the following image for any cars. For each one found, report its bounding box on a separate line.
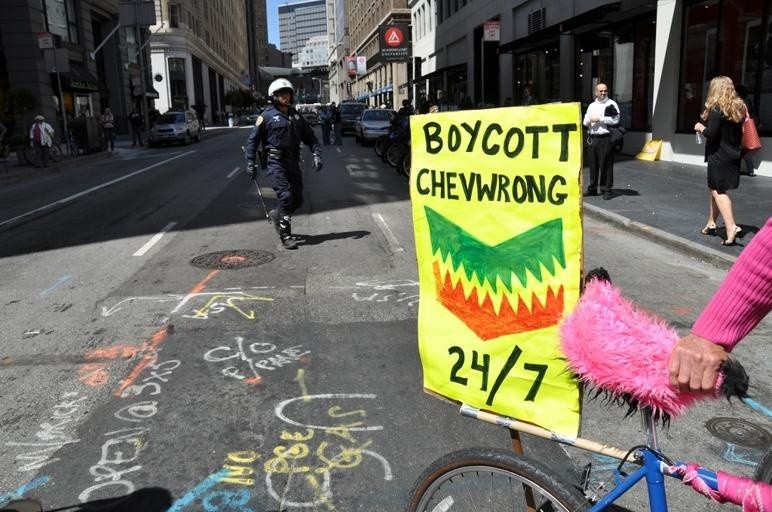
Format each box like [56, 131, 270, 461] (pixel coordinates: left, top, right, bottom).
[352, 107, 400, 149]
[238, 111, 262, 127]
[298, 104, 339, 130]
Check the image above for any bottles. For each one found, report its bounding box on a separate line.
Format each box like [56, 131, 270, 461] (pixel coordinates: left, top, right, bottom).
[694, 130, 703, 145]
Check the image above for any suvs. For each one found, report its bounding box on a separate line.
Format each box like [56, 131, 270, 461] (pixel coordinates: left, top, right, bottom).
[336, 101, 371, 136]
[147, 111, 203, 150]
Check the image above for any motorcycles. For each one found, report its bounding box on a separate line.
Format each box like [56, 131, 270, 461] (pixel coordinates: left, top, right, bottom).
[374, 111, 412, 177]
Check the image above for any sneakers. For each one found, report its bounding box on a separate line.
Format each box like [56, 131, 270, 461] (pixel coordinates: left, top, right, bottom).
[270, 210, 298, 250]
[583, 188, 598, 197]
[603, 191, 613, 200]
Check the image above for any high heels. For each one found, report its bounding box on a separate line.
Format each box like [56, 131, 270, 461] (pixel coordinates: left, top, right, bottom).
[700, 224, 717, 236]
[720, 226, 744, 247]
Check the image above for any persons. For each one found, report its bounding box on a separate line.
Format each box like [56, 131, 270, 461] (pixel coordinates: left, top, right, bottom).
[668, 216, 772, 393]
[319, 101, 343, 146]
[693, 75, 747, 246]
[29, 115, 54, 167]
[244, 78, 324, 249]
[392, 87, 539, 116]
[582, 83, 621, 200]
[99, 108, 114, 150]
[128, 107, 144, 147]
[734, 85, 754, 176]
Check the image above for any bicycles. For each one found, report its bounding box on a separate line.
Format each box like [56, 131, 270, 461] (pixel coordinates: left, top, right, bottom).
[400, 270, 772, 512]
[22, 135, 66, 169]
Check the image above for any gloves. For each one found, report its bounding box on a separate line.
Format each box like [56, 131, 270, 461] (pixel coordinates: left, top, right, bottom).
[311, 153, 323, 173]
[245, 162, 256, 184]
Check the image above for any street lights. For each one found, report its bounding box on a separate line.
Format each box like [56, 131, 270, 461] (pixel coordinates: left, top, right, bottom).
[311, 77, 324, 104]
[336, 41, 361, 102]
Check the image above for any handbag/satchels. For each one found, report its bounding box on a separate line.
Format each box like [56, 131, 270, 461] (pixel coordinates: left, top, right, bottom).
[742, 102, 764, 160]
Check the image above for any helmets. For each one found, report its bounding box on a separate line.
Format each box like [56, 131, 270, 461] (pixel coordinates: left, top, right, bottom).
[267, 77, 294, 107]
